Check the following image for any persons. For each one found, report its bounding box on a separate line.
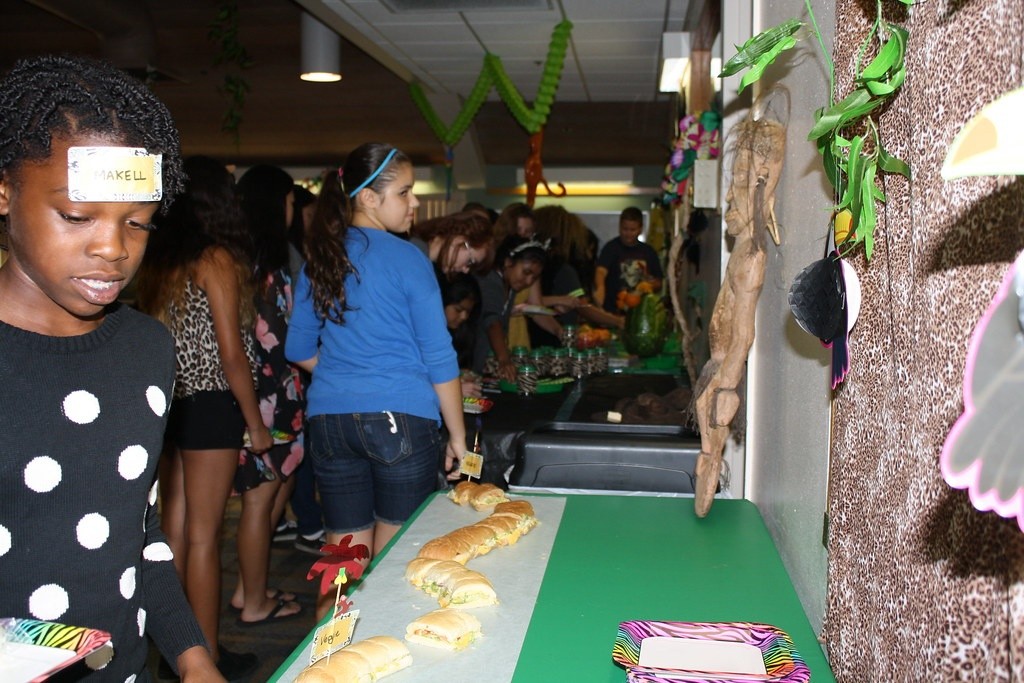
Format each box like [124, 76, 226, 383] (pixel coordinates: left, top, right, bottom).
[119, 144, 709, 683]
[0, 53, 225, 683]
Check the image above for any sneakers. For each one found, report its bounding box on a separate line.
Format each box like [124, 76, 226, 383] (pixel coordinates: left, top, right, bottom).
[272, 521, 299, 542]
[293, 531, 328, 555]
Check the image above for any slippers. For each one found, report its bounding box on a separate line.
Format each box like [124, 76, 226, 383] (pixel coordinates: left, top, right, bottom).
[227, 590, 298, 612]
[236, 599, 306, 628]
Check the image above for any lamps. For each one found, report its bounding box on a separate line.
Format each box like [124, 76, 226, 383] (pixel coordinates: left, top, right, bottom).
[659, 31, 689, 93]
[300, 8, 341, 82]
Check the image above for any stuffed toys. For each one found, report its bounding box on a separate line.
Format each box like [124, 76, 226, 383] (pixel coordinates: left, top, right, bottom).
[788, 249, 861, 389]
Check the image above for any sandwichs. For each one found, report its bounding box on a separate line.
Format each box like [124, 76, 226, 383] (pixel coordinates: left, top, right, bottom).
[404, 481, 537, 610]
[293, 635, 413, 682]
[404, 611, 483, 652]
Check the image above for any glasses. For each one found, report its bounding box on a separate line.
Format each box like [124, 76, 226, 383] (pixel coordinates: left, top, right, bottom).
[463, 240, 474, 266]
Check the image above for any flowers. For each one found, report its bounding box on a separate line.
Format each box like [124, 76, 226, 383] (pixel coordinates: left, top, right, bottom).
[662, 108, 718, 204]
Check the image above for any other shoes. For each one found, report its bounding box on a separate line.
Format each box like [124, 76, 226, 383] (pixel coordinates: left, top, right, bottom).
[214, 646, 261, 681]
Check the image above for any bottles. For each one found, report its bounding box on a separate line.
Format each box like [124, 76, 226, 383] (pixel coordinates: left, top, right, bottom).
[484, 325, 608, 399]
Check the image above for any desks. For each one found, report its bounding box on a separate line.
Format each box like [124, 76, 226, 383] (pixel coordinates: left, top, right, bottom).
[267, 487, 839, 683]
